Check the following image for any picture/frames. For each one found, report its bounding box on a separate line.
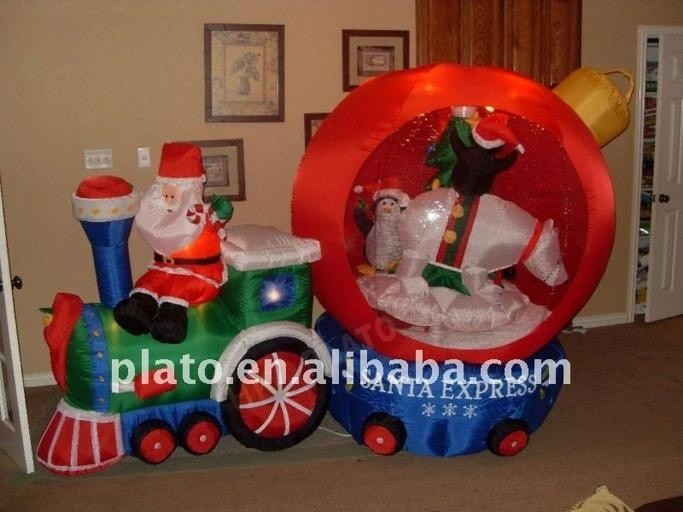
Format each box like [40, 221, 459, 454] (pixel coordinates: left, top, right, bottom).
[195, 21, 412, 207]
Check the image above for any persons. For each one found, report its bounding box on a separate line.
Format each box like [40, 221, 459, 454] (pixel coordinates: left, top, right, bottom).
[33, 60, 637, 479]
